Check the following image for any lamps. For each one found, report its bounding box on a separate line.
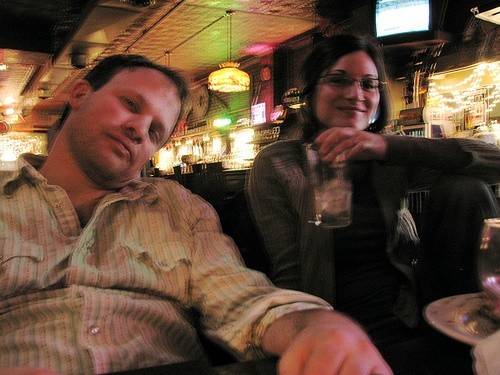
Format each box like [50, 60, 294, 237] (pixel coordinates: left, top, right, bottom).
[207, 10, 250, 94]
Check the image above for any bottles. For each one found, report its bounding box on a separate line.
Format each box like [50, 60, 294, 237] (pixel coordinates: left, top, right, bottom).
[283, 88, 301, 105]
[187, 120, 208, 133]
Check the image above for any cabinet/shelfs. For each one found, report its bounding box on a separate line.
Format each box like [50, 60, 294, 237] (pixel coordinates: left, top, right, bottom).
[242, 123, 282, 162]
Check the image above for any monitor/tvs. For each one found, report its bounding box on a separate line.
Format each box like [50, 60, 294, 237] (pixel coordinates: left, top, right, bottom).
[375, 0, 439, 45]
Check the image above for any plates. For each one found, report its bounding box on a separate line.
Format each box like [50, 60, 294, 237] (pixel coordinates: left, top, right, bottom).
[424, 292, 500, 346]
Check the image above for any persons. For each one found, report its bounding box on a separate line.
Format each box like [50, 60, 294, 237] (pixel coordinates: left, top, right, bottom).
[0, 53, 394, 375]
[248, 31, 500, 350]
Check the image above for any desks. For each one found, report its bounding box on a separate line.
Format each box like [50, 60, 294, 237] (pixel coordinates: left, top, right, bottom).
[425, 292, 500, 346]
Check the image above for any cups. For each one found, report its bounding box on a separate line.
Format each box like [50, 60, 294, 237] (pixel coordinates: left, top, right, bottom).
[304, 144, 354, 229]
[477, 217, 500, 323]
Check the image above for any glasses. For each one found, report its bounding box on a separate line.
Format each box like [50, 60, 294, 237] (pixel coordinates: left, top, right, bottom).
[317, 73, 387, 91]
